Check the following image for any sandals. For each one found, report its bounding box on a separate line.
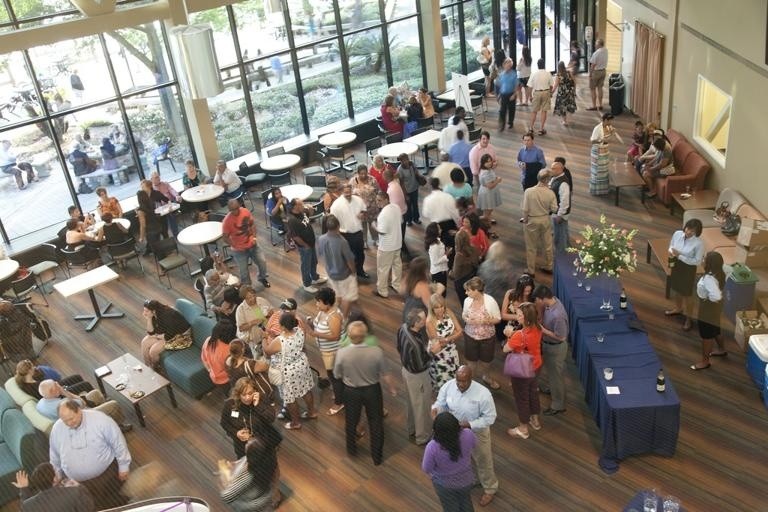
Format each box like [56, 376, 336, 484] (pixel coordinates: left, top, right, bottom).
[527, 129, 534, 134]
[538, 129, 546, 136]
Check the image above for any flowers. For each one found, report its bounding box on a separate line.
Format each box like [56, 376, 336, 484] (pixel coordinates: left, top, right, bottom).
[563, 212, 641, 281]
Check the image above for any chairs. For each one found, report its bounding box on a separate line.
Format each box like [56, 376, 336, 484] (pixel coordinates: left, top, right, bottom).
[0, 386, 21, 443]
[1, 179, 230, 336]
[2, 104, 149, 188]
[229, 79, 484, 238]
[163, 315, 218, 401]
[158, 296, 208, 372]
[21, 398, 55, 440]
[149, 136, 179, 175]
[4, 376, 39, 405]
[0, 408, 43, 508]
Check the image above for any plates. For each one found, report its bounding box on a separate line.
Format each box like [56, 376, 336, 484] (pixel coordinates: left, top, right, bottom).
[131, 391, 145, 398]
[115, 383, 126, 390]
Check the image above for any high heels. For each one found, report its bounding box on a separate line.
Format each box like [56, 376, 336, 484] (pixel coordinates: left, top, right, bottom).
[503, 320, 514, 338]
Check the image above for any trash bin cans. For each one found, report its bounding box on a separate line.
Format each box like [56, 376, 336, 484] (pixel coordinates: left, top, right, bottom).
[441, 13, 449, 37]
[723, 262, 760, 324]
[608, 73, 625, 115]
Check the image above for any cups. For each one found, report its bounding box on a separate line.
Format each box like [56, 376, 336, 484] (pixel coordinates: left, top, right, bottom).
[595, 332, 605, 342]
[505, 325, 514, 337]
[642, 486, 658, 512]
[576, 280, 583, 287]
[602, 367, 614, 380]
[603, 297, 615, 320]
[586, 286, 591, 292]
[241, 428, 250, 438]
[662, 494, 681, 512]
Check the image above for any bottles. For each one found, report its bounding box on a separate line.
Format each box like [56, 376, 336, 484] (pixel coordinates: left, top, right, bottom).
[656, 368, 666, 392]
[619, 287, 627, 309]
[740, 309, 759, 329]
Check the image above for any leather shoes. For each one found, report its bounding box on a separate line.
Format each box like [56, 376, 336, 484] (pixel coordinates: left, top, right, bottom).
[539, 265, 553, 274]
[479, 492, 497, 507]
[638, 185, 658, 199]
[523, 268, 535, 278]
[520, 217, 524, 222]
[543, 407, 566, 416]
[586, 107, 597, 110]
[490, 218, 496, 224]
[508, 124, 513, 128]
[538, 384, 551, 395]
[357, 270, 369, 278]
[356, 428, 367, 441]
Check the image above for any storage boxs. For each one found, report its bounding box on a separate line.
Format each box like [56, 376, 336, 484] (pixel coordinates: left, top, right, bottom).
[733, 310, 768, 352]
[745, 332, 768, 389]
[734, 214, 768, 271]
[761, 362, 768, 409]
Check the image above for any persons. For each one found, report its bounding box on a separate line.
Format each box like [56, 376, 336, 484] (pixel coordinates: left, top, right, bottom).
[590, 112, 624, 197]
[381, 87, 436, 139]
[664, 218, 728, 370]
[627, 120, 674, 198]
[3, 49, 280, 261]
[140, 263, 386, 488]
[10, 359, 132, 512]
[478, 9, 580, 139]
[585, 38, 608, 110]
[395, 107, 572, 511]
[265, 155, 409, 318]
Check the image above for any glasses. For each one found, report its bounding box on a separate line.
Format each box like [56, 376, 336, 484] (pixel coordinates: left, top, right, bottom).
[145, 298, 152, 304]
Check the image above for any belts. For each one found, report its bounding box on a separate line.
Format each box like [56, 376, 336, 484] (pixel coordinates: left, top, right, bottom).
[543, 340, 561, 345]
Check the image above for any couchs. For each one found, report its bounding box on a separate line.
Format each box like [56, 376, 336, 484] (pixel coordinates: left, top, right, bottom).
[655, 128, 711, 208]
[682, 186, 768, 295]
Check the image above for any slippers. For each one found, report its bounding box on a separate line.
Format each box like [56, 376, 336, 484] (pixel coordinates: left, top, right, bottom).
[507, 427, 530, 440]
[483, 374, 500, 390]
[528, 415, 542, 431]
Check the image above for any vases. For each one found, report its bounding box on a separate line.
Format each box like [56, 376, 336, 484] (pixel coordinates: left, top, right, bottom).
[600, 278, 614, 312]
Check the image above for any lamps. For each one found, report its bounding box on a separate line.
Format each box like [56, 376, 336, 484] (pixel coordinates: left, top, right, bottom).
[166, 0, 225, 103]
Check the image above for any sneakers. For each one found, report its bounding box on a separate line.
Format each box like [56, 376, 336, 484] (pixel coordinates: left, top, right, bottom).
[373, 239, 379, 246]
[285, 239, 296, 249]
[665, 308, 728, 371]
[311, 277, 328, 285]
[258, 279, 270, 288]
[364, 240, 368, 249]
[406, 220, 412, 226]
[413, 218, 422, 224]
[277, 401, 344, 430]
[303, 285, 319, 293]
[119, 423, 132, 433]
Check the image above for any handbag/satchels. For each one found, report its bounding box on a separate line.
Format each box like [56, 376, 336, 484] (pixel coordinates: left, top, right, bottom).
[721, 211, 742, 236]
[659, 165, 676, 176]
[163, 329, 193, 351]
[477, 46, 489, 64]
[713, 201, 731, 224]
[411, 163, 427, 186]
[504, 330, 536, 379]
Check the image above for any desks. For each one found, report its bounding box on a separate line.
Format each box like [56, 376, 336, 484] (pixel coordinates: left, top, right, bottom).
[670, 188, 720, 216]
[553, 253, 635, 359]
[609, 161, 648, 207]
[574, 311, 682, 474]
[622, 487, 689, 511]
[646, 238, 706, 299]
[756, 295, 767, 316]
[93, 352, 178, 428]
